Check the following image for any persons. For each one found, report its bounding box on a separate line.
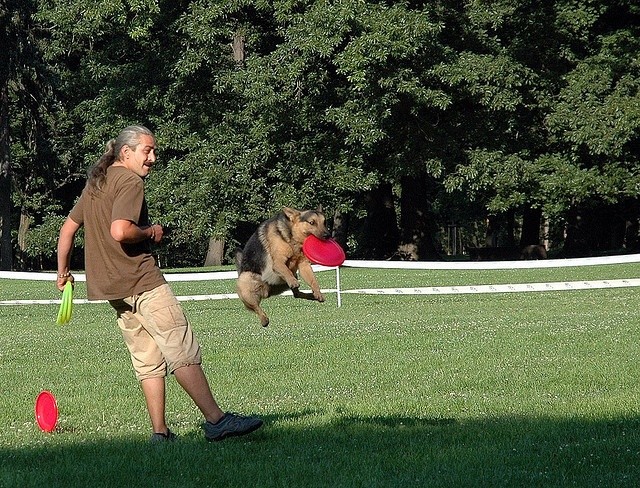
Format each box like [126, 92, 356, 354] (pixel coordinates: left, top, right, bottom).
[57, 124, 264, 446]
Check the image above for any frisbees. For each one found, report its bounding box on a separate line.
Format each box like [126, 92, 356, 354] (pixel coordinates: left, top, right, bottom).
[55, 279, 74, 325]
[35, 391, 57, 433]
[302, 235, 346, 267]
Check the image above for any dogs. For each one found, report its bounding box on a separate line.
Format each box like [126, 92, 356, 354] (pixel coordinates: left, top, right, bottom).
[233, 202, 332, 328]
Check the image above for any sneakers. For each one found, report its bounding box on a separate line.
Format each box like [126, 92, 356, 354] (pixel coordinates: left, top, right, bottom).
[203, 411, 263, 442]
[153, 428, 176, 442]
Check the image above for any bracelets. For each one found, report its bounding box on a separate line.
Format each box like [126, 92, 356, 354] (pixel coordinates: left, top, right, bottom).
[57, 270, 71, 278]
[149, 224, 155, 239]
